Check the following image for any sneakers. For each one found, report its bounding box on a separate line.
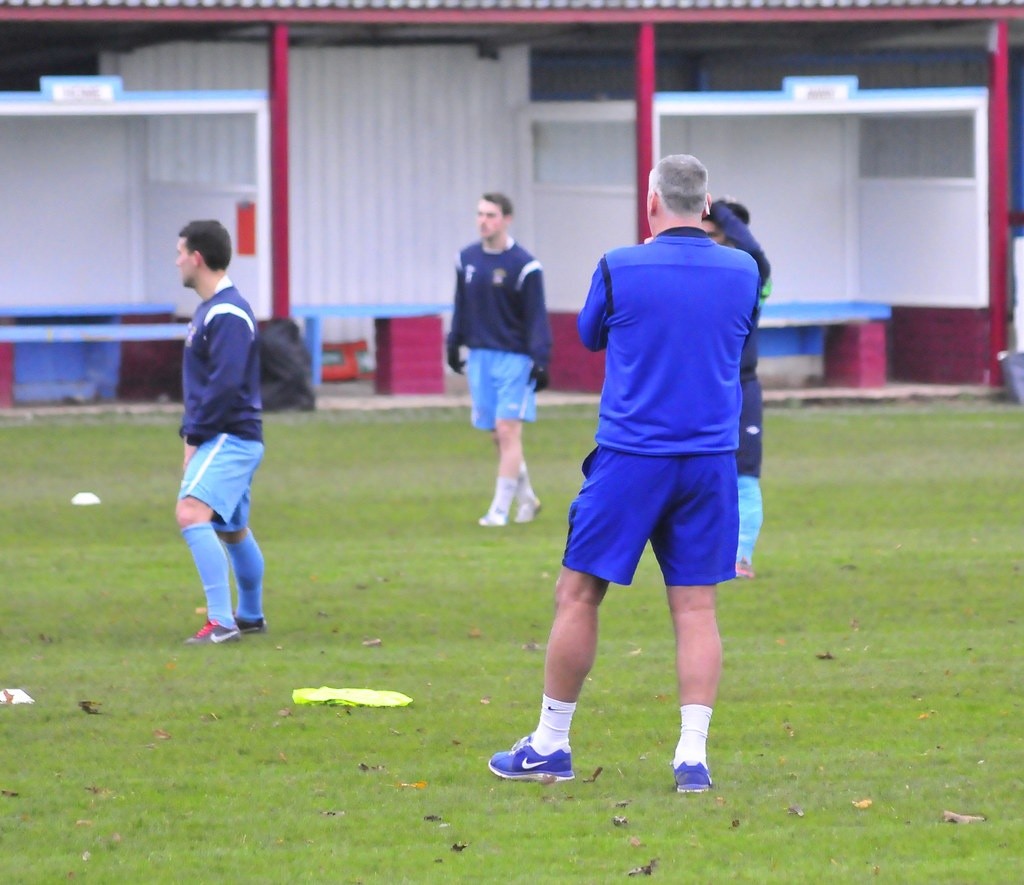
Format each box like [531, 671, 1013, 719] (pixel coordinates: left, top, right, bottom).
[674, 761, 712, 793]
[235, 613, 267, 632]
[185, 620, 243, 646]
[488, 737, 575, 782]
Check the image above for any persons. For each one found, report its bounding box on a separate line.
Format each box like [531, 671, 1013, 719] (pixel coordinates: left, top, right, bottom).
[701, 197, 771, 579]
[174, 220, 266, 646]
[486, 154, 760, 793]
[446, 193, 552, 526]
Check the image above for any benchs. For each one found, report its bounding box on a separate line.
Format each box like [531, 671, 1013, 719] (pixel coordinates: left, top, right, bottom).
[293, 303, 454, 393]
[0, 303, 190, 417]
[757, 303, 893, 392]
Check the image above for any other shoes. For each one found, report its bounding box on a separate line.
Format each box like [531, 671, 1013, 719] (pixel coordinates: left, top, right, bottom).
[479, 506, 510, 527]
[515, 497, 541, 525]
